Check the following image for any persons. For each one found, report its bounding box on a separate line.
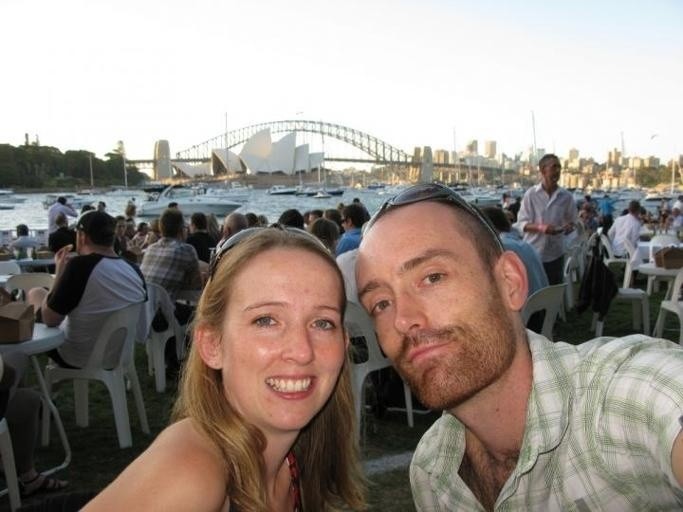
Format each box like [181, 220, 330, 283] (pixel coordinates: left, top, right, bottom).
[579, 191, 683, 259]
[76, 222, 347, 510]
[10, 198, 388, 363]
[517, 155, 577, 335]
[355, 182, 683, 512]
[0, 350, 70, 497]
[499, 190, 521, 223]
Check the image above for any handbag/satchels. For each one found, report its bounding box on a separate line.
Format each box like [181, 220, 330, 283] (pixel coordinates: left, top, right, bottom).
[591, 257, 617, 320]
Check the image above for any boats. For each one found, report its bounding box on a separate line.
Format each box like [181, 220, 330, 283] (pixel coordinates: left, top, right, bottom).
[44, 194, 96, 211]
[0, 189, 27, 207]
[133, 185, 240, 218]
[266, 182, 346, 198]
[107, 188, 142, 198]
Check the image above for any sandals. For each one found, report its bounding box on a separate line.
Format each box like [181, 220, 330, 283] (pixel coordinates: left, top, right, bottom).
[20, 472, 71, 498]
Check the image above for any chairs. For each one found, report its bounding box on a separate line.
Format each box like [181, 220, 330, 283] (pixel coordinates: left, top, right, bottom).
[0, 228, 683, 512]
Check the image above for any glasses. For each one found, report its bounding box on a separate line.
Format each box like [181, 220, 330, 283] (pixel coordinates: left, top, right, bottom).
[359, 183, 505, 253]
[209, 225, 330, 283]
